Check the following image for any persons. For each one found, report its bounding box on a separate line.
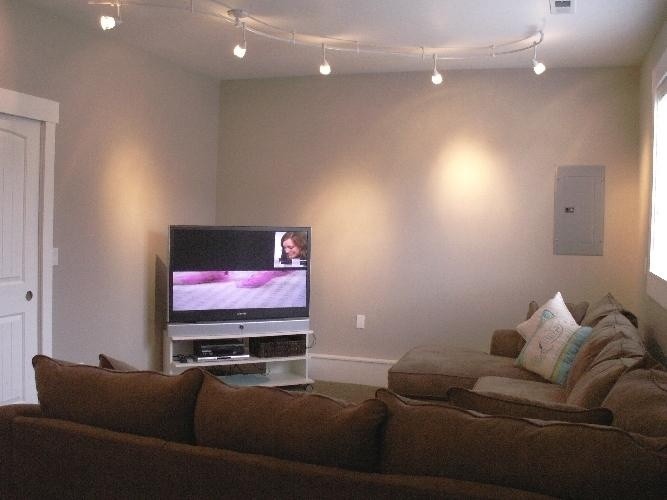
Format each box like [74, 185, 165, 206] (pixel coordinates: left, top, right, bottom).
[278, 231, 306, 266]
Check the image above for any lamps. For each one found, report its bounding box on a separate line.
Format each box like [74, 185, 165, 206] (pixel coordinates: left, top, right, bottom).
[87, 0, 546, 85]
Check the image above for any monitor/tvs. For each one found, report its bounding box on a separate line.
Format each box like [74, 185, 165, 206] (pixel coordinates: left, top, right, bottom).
[167, 224, 311, 338]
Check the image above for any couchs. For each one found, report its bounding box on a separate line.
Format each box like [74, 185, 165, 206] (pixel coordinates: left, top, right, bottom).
[0, 330, 667, 500]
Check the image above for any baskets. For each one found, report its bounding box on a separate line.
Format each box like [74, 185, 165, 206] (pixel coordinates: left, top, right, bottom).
[249, 336, 305, 357]
